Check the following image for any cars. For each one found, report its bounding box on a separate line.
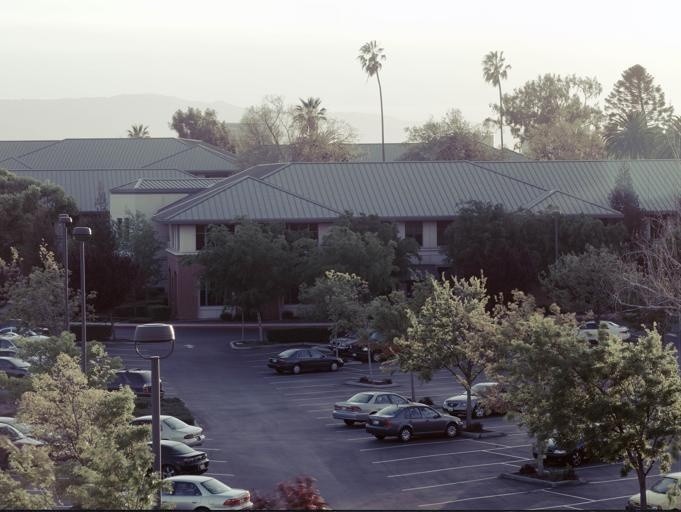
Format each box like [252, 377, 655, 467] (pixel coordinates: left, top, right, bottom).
[116, 369, 164, 397]
[578, 320, 630, 344]
[0, 326, 49, 377]
[0, 416, 61, 469]
[533, 423, 603, 466]
[130, 415, 253, 512]
[332, 382, 502, 441]
[268, 330, 381, 374]
[626, 472, 681, 511]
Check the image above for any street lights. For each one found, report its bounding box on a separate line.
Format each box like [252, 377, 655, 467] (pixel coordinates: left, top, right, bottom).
[134, 323, 177, 510]
[57, 212, 72, 332]
[73, 227, 93, 375]
